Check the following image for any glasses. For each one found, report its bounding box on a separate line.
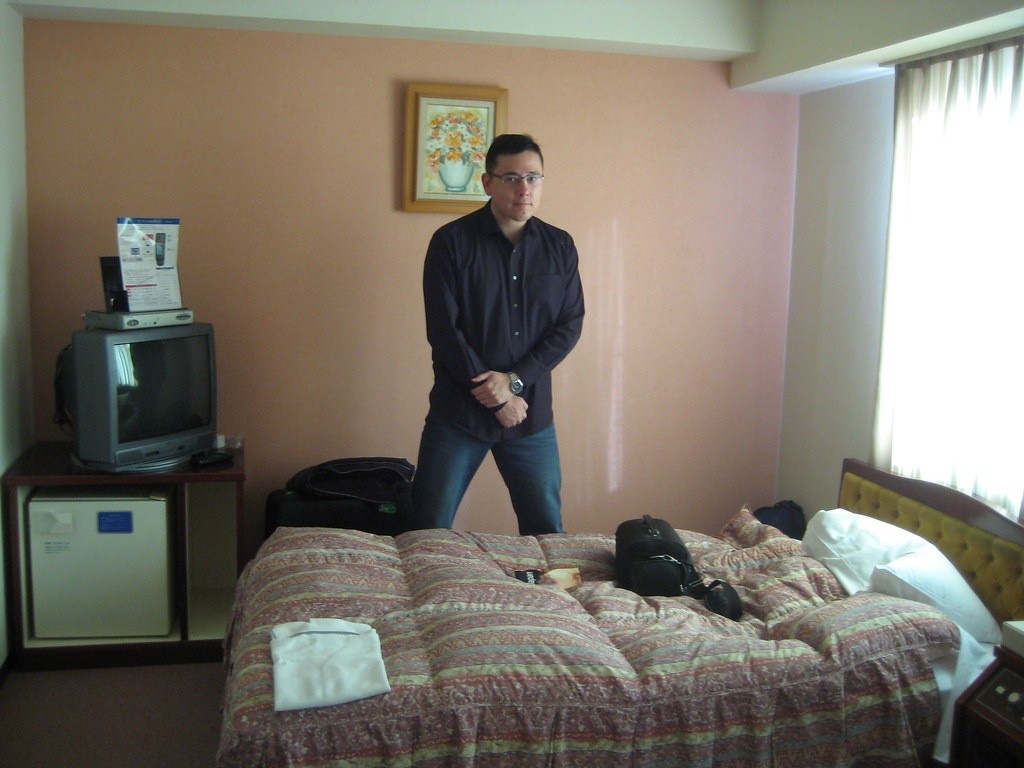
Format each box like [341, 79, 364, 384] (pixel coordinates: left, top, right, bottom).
[489, 172, 545, 186]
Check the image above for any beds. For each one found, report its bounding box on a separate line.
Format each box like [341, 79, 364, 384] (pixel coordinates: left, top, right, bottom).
[222, 455, 1024, 768]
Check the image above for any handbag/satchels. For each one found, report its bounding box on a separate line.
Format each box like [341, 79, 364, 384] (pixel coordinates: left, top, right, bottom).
[615, 516, 742, 622]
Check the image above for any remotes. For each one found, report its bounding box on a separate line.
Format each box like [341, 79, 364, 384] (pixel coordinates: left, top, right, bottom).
[197, 454, 234, 465]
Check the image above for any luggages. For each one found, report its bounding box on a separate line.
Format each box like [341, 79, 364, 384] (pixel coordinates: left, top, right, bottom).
[266, 456, 415, 536]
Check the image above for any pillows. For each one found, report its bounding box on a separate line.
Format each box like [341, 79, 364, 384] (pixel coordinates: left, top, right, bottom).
[804, 507, 1004, 644]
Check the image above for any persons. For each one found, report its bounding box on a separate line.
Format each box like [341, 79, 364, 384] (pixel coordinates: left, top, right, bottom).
[413, 134, 585, 534]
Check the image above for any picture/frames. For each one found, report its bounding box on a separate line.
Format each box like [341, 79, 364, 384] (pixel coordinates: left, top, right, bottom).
[403, 81, 508, 213]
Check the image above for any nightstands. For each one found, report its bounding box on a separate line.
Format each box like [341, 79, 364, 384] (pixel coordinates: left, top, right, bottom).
[947, 645, 1024, 768]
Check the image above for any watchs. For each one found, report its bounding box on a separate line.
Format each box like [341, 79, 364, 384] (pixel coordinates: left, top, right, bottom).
[508, 372, 523, 394]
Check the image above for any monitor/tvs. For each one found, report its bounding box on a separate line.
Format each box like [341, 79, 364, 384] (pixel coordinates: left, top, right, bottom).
[55, 322, 221, 472]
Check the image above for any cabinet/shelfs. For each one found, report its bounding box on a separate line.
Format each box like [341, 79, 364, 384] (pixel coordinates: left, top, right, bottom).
[0, 439, 247, 667]
